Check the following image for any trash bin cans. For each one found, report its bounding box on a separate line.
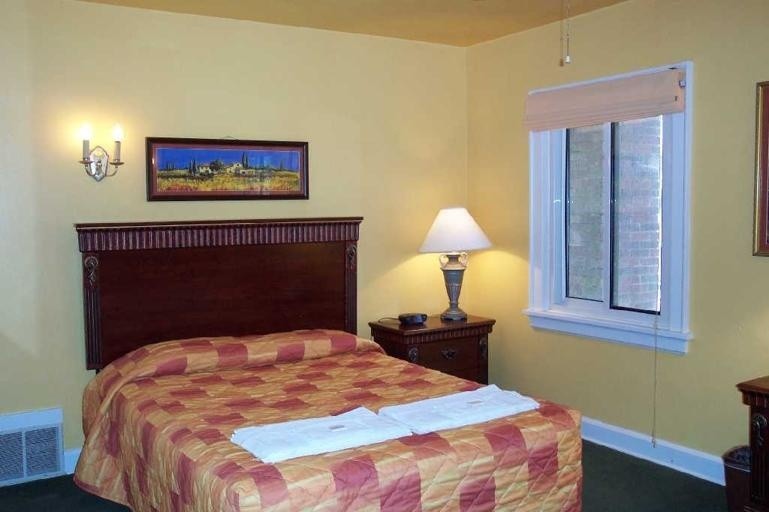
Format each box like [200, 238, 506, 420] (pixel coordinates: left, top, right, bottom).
[721, 445, 751, 512]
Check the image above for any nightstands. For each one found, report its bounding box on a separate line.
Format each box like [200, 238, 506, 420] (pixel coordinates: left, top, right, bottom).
[369, 311, 495, 387]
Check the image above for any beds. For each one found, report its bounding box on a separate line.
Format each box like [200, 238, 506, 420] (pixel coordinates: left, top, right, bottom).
[77, 217, 583, 512]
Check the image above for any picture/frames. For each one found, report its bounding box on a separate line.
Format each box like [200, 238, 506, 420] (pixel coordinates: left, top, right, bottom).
[145, 136, 309, 201]
[753, 80, 769, 257]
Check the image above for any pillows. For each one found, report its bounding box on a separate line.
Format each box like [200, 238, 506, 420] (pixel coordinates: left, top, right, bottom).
[120, 330, 376, 383]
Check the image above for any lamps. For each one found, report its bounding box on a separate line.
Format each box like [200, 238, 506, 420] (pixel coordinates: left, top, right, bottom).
[420, 207, 493, 323]
[78, 123, 125, 181]
